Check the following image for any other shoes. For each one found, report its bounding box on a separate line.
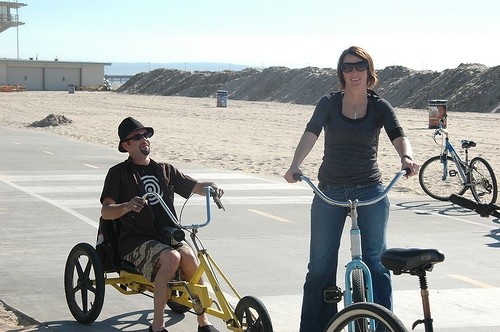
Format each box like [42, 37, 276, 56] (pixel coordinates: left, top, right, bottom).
[198, 325, 219, 332]
[149, 325, 168, 332]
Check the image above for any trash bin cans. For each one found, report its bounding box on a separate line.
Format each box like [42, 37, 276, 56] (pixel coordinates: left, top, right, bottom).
[428, 100, 447, 129]
[68, 83, 75, 94]
[217, 90, 228, 107]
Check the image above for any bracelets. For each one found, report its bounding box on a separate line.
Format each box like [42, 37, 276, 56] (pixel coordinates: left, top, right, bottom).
[401, 155, 412, 161]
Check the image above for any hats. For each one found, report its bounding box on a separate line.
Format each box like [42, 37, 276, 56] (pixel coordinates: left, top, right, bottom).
[118, 117, 154, 152]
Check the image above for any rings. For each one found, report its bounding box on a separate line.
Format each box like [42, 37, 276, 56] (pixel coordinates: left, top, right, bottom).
[133, 205, 137, 208]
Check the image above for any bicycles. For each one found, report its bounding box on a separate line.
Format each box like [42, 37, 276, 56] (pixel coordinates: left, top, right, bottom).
[292, 167, 445, 332]
[419, 116, 498, 207]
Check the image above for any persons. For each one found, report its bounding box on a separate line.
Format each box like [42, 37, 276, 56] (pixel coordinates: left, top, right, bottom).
[285, 47, 419, 332]
[100, 118, 224, 332]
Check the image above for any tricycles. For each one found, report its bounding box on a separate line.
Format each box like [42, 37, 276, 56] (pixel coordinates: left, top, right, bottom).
[63, 186, 273, 332]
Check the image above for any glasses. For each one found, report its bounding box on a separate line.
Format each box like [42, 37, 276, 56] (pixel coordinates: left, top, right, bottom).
[341, 60, 368, 73]
[121, 131, 152, 142]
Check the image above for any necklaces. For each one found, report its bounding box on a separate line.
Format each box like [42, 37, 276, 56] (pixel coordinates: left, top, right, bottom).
[344, 96, 367, 119]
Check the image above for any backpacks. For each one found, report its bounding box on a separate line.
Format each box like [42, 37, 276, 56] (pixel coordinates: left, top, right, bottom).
[96, 216, 123, 272]
